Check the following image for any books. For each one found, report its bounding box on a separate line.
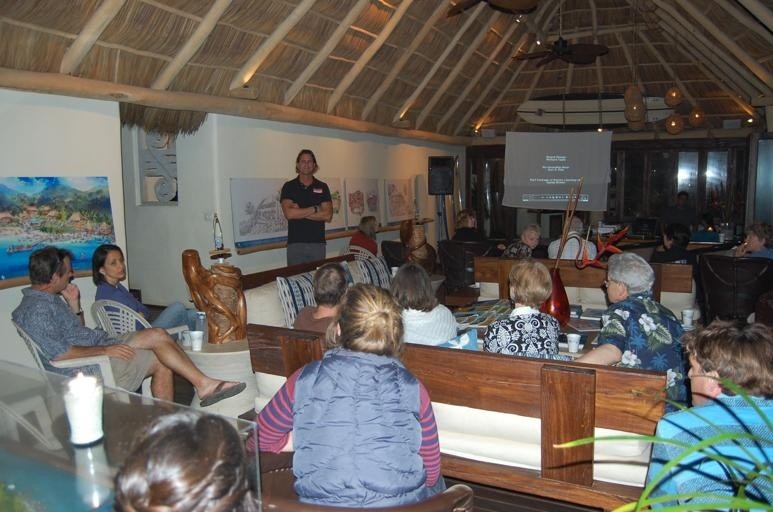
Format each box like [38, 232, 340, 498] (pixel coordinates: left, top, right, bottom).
[453, 298, 513, 328]
[567, 319, 603, 332]
[579, 307, 607, 321]
[569, 304, 583, 318]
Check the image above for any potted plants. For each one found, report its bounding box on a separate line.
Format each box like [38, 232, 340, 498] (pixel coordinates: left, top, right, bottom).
[539, 181, 629, 327]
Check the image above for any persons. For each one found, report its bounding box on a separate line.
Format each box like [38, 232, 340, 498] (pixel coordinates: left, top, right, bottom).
[92, 243, 197, 342]
[351, 215, 379, 259]
[732, 221, 773, 260]
[642, 315, 772, 512]
[450, 208, 504, 257]
[571, 252, 690, 415]
[279, 149, 335, 268]
[547, 215, 596, 261]
[292, 261, 349, 338]
[111, 407, 255, 511]
[388, 262, 457, 348]
[483, 260, 561, 360]
[648, 223, 698, 266]
[244, 282, 447, 509]
[501, 223, 541, 258]
[659, 191, 713, 240]
[10, 246, 247, 411]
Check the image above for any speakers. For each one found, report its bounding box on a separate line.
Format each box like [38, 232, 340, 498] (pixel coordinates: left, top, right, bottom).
[427, 156, 453, 195]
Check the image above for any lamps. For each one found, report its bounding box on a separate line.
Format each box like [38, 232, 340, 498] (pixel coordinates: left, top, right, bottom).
[625, 7, 644, 105]
[665, 111, 685, 137]
[665, 33, 682, 107]
[689, 62, 707, 129]
[628, 118, 645, 131]
[625, 14, 645, 121]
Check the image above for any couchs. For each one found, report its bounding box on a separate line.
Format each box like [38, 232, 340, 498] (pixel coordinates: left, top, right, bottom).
[473, 255, 700, 322]
[239, 254, 359, 328]
[245, 323, 667, 509]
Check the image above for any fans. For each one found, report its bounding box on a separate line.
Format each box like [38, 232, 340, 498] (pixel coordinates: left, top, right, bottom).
[512, 4, 609, 68]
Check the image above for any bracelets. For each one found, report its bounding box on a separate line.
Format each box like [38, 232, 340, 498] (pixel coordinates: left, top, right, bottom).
[312, 205, 318, 213]
[75, 307, 84, 316]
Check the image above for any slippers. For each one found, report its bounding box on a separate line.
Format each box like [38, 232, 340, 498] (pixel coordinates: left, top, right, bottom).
[200, 380, 246, 407]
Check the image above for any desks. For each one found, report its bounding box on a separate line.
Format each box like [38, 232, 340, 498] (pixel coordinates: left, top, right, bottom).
[456, 302, 702, 360]
[0, 359, 263, 510]
[176, 339, 256, 418]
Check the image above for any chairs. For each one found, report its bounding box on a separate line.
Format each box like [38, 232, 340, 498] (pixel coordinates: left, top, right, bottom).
[381, 240, 436, 274]
[90, 299, 190, 404]
[243, 449, 474, 511]
[699, 254, 773, 323]
[438, 240, 496, 289]
[343, 245, 375, 260]
[9, 319, 128, 405]
[0, 399, 63, 451]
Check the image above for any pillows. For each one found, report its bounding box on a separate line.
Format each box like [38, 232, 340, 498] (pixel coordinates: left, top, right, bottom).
[277, 260, 349, 329]
[354, 254, 392, 290]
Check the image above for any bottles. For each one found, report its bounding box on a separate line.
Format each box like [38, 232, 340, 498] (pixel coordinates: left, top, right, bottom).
[195, 315, 209, 346]
[214, 218, 223, 250]
[63, 372, 105, 446]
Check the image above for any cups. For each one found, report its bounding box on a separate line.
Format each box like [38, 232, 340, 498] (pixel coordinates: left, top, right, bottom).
[182, 330, 193, 347]
[719, 233, 725, 242]
[390, 267, 400, 278]
[566, 334, 582, 353]
[682, 310, 693, 326]
[189, 331, 204, 352]
